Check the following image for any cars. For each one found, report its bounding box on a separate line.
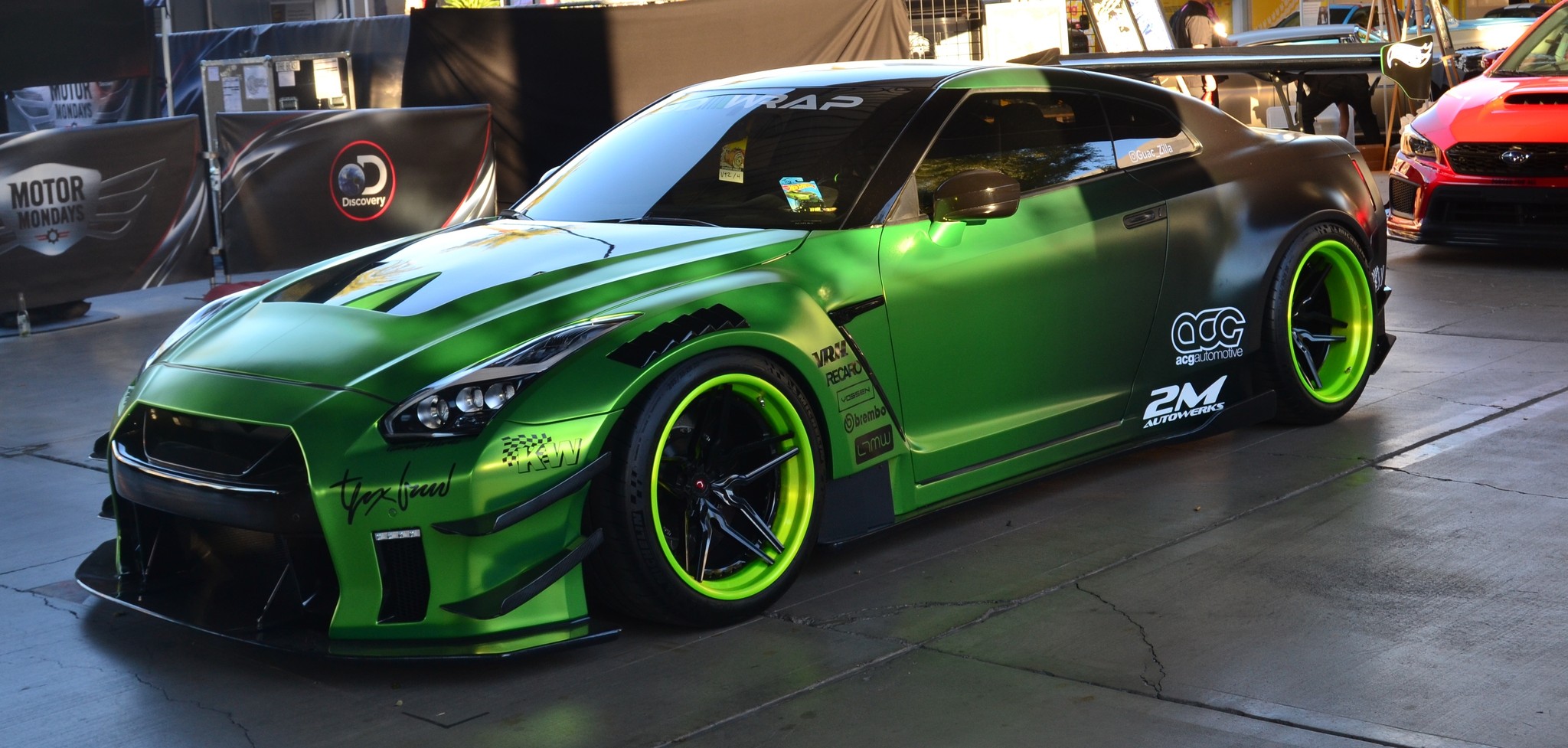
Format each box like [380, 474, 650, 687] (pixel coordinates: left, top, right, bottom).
[1386, 1, 1568, 264]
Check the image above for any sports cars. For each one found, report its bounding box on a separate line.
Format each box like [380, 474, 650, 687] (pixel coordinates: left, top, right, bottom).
[74, 39, 1409, 663]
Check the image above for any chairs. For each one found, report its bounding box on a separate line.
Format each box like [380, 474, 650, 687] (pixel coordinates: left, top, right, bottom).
[990, 101, 1050, 149]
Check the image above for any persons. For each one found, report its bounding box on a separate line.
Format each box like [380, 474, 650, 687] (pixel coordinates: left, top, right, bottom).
[1169, 0, 1241, 112]
[1282, 67, 1383, 145]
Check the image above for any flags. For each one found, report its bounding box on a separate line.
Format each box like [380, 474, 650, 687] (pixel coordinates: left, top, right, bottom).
[1380, 34, 1433, 103]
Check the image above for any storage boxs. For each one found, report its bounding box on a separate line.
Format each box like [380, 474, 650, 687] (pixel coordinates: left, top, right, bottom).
[1357, 144, 1386, 172]
